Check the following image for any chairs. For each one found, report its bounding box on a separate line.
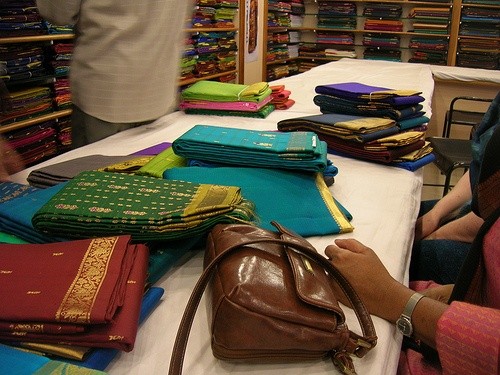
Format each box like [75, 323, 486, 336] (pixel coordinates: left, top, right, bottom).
[441, 95, 495, 139]
[425, 135, 474, 197]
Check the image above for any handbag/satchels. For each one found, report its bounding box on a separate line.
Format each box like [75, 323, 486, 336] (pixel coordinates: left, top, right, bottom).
[168, 219, 378, 375]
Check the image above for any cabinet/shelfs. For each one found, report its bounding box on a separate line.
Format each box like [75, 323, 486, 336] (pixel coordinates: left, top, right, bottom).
[176, 26, 239, 87]
[298, 0, 500, 67]
[0, 34, 78, 135]
[267, 26, 299, 82]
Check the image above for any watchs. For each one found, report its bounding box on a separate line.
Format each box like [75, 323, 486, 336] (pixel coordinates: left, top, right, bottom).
[396, 292, 426, 338]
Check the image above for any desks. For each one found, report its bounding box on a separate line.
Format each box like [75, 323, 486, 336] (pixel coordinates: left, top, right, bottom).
[0, 59, 435, 375]
[426, 61, 500, 140]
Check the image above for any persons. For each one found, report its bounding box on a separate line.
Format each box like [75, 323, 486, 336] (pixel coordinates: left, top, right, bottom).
[323, 204, 500, 375]
[37, 0, 190, 150]
[406, 87, 500, 287]
